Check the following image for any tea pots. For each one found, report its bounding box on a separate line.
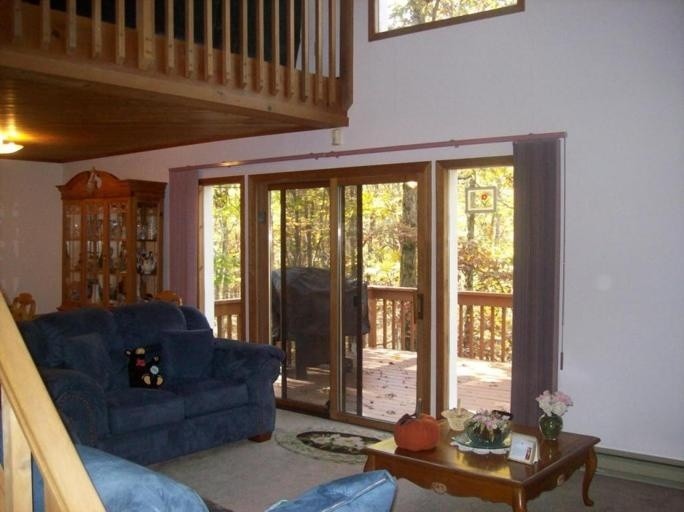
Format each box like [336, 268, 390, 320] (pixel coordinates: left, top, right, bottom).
[140, 259, 156, 275]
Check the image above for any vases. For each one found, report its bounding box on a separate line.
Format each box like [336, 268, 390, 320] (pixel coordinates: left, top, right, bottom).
[538, 412, 563, 441]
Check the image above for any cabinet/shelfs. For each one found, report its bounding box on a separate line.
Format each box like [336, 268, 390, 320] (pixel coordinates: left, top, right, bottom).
[54, 170, 165, 312]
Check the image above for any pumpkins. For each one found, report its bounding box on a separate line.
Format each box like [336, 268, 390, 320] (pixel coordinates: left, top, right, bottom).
[394, 397, 440, 452]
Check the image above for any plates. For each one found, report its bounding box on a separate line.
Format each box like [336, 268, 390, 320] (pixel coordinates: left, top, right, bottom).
[455, 432, 512, 449]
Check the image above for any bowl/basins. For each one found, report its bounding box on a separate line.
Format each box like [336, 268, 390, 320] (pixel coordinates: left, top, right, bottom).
[463, 417, 512, 444]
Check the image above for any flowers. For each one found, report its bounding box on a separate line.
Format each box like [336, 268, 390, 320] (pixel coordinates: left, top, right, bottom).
[535, 390, 574, 417]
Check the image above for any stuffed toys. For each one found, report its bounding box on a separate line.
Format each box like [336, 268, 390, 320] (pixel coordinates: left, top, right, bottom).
[124, 346, 165, 388]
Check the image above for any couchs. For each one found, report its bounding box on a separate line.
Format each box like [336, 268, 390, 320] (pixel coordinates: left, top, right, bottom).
[16, 305, 285, 467]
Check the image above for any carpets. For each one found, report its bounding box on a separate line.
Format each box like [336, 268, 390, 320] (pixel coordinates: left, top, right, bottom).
[274, 425, 391, 464]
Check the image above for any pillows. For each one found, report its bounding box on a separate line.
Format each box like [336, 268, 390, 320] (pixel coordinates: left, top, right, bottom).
[154, 328, 214, 388]
[61, 332, 112, 390]
